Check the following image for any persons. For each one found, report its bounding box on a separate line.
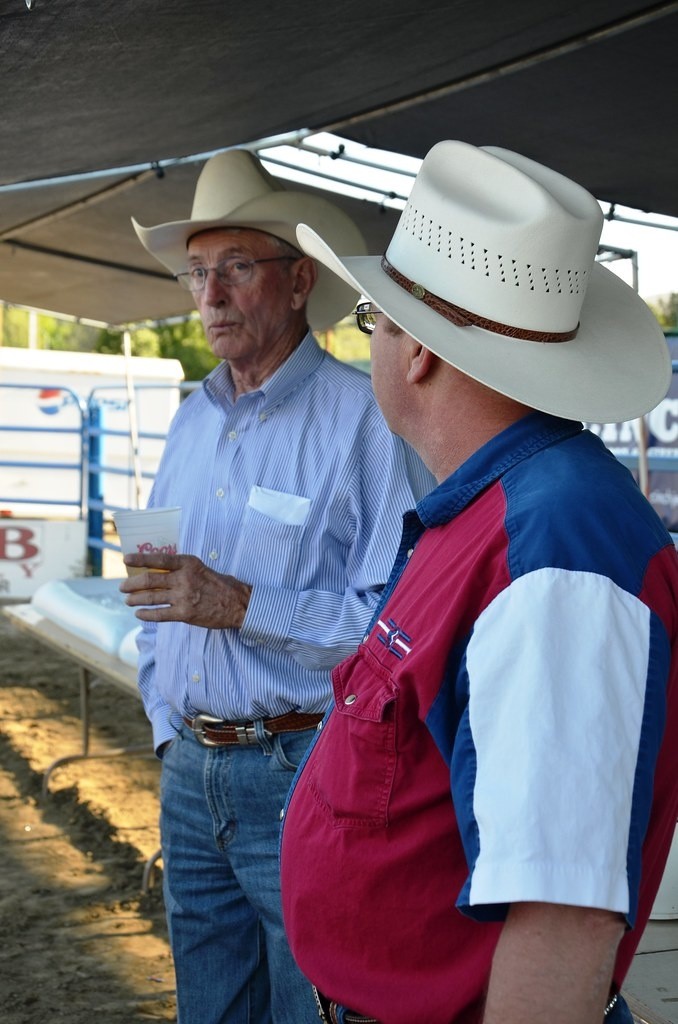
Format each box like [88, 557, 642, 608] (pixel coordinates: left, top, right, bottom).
[118, 148, 439, 1024]
[281, 140, 678, 1024]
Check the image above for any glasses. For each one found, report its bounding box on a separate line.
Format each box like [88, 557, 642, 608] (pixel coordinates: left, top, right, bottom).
[353, 303, 384, 335]
[172, 256, 300, 290]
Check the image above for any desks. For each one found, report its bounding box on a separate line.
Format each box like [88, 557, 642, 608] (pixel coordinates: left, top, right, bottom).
[1, 603, 144, 755]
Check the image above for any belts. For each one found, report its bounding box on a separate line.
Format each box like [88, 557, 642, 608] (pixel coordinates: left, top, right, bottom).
[311, 979, 618, 1024]
[180, 711, 325, 747]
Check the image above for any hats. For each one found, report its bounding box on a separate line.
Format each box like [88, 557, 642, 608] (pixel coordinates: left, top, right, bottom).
[129, 148, 369, 329]
[296, 138, 671, 423]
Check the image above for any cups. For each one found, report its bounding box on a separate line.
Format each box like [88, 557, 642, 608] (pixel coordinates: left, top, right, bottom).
[110, 505, 183, 594]
